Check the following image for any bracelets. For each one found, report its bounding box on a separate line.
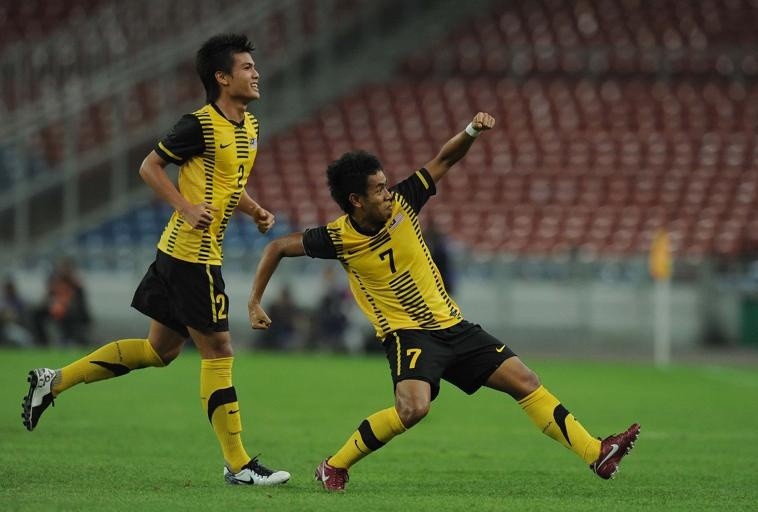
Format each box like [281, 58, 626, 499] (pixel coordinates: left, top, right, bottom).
[465, 121, 482, 139]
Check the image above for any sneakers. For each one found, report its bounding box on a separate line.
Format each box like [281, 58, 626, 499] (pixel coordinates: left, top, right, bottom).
[589, 423, 642, 480]
[223, 458, 291, 485]
[314, 455, 349, 490]
[21, 367, 57, 432]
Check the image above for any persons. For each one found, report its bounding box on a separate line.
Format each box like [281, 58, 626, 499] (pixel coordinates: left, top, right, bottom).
[254, 287, 298, 351]
[306, 264, 352, 356]
[26, 270, 94, 346]
[0, 276, 35, 347]
[246, 111, 643, 494]
[420, 215, 452, 298]
[22, 31, 294, 487]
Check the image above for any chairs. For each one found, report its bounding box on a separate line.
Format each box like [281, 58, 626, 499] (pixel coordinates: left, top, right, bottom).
[1, 1, 758, 264]
